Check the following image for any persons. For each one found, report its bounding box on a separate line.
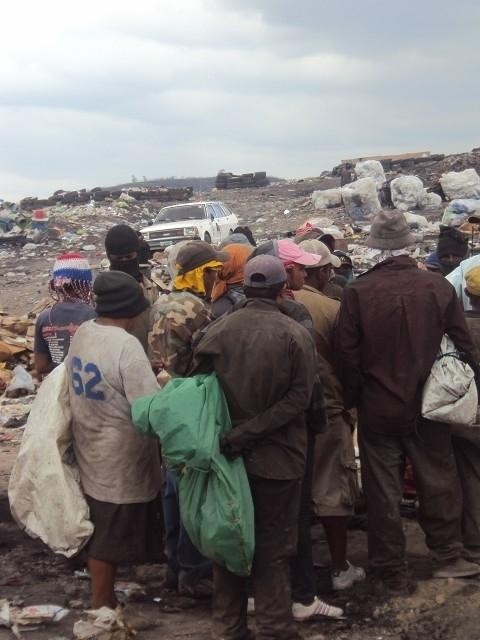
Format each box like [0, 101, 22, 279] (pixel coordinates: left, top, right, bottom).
[33, 207, 480, 640]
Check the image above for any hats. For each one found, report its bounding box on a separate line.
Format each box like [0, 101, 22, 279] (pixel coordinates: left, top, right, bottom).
[93, 272, 150, 317]
[277, 239, 322, 271]
[51, 254, 92, 281]
[465, 266, 480, 296]
[176, 240, 228, 275]
[437, 225, 468, 276]
[298, 239, 341, 269]
[295, 228, 335, 253]
[243, 254, 289, 289]
[105, 224, 143, 282]
[365, 209, 416, 250]
[338, 255, 354, 268]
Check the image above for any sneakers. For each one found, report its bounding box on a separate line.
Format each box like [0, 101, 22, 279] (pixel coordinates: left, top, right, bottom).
[331, 560, 365, 590]
[433, 557, 479, 578]
[387, 569, 417, 594]
[177, 571, 215, 602]
[87, 605, 149, 630]
[291, 595, 343, 621]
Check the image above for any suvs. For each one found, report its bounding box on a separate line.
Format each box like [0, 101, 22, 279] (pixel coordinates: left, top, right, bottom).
[138, 201, 239, 259]
